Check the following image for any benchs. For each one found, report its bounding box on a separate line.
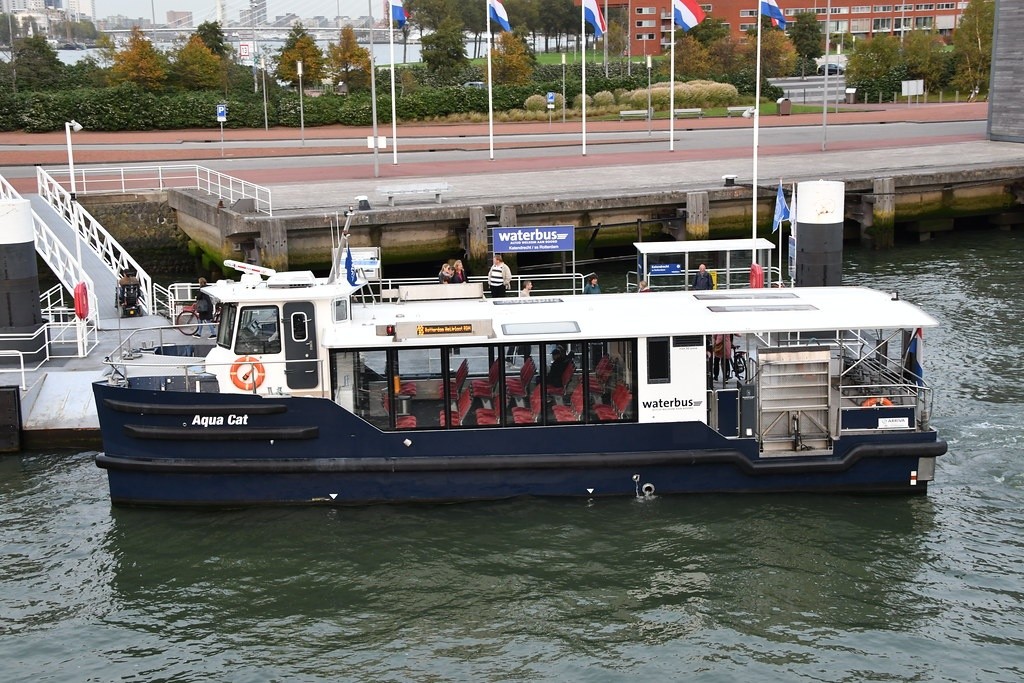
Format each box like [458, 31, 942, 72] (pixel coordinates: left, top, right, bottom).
[387, 354, 632, 427]
[674, 108, 704, 120]
[727, 106, 755, 117]
[618, 109, 648, 122]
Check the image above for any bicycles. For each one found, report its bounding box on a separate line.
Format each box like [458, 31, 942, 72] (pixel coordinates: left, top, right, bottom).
[177, 299, 235, 336]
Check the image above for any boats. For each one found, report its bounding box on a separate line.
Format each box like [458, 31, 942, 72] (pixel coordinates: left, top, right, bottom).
[91, 205, 945, 509]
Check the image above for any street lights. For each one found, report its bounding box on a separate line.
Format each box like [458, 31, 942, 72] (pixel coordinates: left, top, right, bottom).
[65, 120, 88, 347]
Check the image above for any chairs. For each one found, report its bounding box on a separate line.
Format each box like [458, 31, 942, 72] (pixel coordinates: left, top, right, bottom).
[844, 356, 896, 404]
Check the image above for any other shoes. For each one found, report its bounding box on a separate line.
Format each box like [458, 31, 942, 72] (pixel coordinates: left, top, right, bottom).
[713, 376, 718, 382]
[193, 334, 200, 338]
[208, 335, 217, 339]
[725, 378, 728, 382]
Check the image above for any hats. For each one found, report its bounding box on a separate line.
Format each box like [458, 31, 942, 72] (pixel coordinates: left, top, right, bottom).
[550, 349, 559, 354]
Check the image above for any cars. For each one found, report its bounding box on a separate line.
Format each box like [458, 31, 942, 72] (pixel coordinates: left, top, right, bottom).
[818, 64, 844, 76]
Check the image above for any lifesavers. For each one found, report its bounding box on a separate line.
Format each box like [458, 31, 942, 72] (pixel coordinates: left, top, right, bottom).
[862, 397, 892, 406]
[73, 281, 89, 319]
[749, 263, 764, 288]
[230, 356, 266, 391]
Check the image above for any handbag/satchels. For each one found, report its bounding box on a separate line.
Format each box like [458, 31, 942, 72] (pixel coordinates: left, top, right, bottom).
[714, 343, 725, 356]
[197, 294, 208, 312]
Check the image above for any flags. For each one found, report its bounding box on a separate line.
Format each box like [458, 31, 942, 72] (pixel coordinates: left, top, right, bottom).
[582, 0, 606, 37]
[759, 0, 786, 30]
[391, 0, 412, 29]
[487, 0, 510, 31]
[672, 0, 706, 31]
[908, 327, 924, 392]
[345, 242, 360, 286]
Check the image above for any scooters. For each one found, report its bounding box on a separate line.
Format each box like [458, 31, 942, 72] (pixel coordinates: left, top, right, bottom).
[115, 283, 143, 318]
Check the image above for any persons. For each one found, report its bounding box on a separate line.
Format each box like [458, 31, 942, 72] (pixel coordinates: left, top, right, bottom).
[488, 254, 511, 297]
[507, 344, 567, 403]
[439, 259, 468, 284]
[639, 281, 651, 292]
[114, 268, 140, 306]
[693, 264, 713, 290]
[712, 333, 741, 383]
[520, 280, 532, 296]
[192, 277, 217, 339]
[584, 274, 601, 294]
